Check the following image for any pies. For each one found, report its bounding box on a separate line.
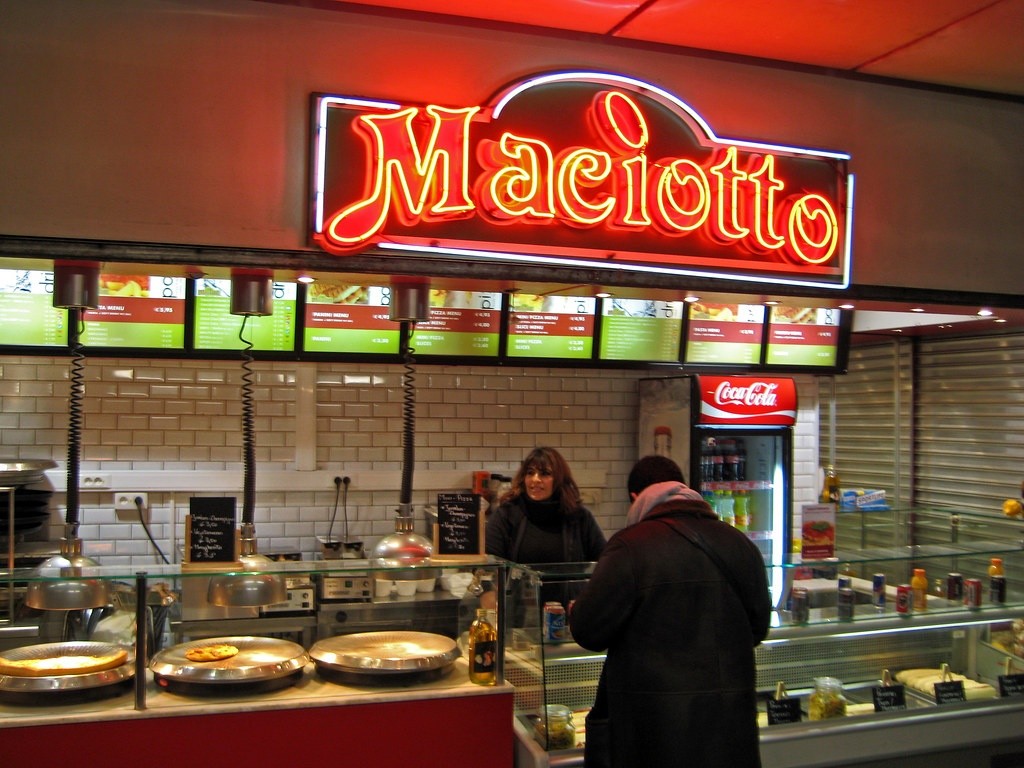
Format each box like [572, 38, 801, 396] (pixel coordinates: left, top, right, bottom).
[185, 645, 238, 662]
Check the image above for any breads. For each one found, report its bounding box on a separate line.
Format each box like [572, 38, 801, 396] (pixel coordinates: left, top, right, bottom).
[895, 668, 997, 700]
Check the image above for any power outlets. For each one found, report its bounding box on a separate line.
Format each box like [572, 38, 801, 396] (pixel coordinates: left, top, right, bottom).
[325, 471, 358, 488]
[79, 474, 112, 489]
[114, 493, 149, 510]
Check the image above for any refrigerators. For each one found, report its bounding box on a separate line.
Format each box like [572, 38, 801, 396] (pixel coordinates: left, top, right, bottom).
[637, 375, 798, 624]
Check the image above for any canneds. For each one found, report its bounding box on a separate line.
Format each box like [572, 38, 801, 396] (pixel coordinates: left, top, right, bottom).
[543, 600, 576, 640]
[872, 573, 886, 608]
[838, 577, 852, 591]
[838, 588, 855, 621]
[791, 587, 810, 623]
[947, 572, 1007, 607]
[896, 584, 913, 616]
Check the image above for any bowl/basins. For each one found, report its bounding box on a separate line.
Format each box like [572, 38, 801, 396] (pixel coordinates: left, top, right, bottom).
[417, 580, 435, 592]
[376, 580, 392, 596]
[396, 580, 416, 596]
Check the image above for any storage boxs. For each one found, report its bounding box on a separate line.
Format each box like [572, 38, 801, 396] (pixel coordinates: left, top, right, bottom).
[839, 487, 892, 512]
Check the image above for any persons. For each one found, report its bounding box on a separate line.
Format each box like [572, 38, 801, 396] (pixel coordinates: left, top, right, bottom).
[570, 454, 772, 768]
[484, 446, 609, 627]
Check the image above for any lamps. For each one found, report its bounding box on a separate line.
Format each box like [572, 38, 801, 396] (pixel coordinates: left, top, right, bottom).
[23, 260, 110, 611]
[206, 269, 286, 606]
[367, 286, 442, 582]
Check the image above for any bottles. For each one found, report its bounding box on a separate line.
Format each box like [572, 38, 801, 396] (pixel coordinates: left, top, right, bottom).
[988, 557, 1006, 603]
[468, 608, 496, 686]
[822, 464, 840, 510]
[911, 569, 928, 611]
[700, 439, 749, 532]
[808, 677, 846, 721]
[0, 460, 57, 537]
[537, 706, 573, 749]
[654, 427, 672, 460]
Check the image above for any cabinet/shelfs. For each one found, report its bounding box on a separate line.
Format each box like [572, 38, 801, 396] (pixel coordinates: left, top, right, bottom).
[504, 536, 1024, 768]
[0, 553, 515, 768]
